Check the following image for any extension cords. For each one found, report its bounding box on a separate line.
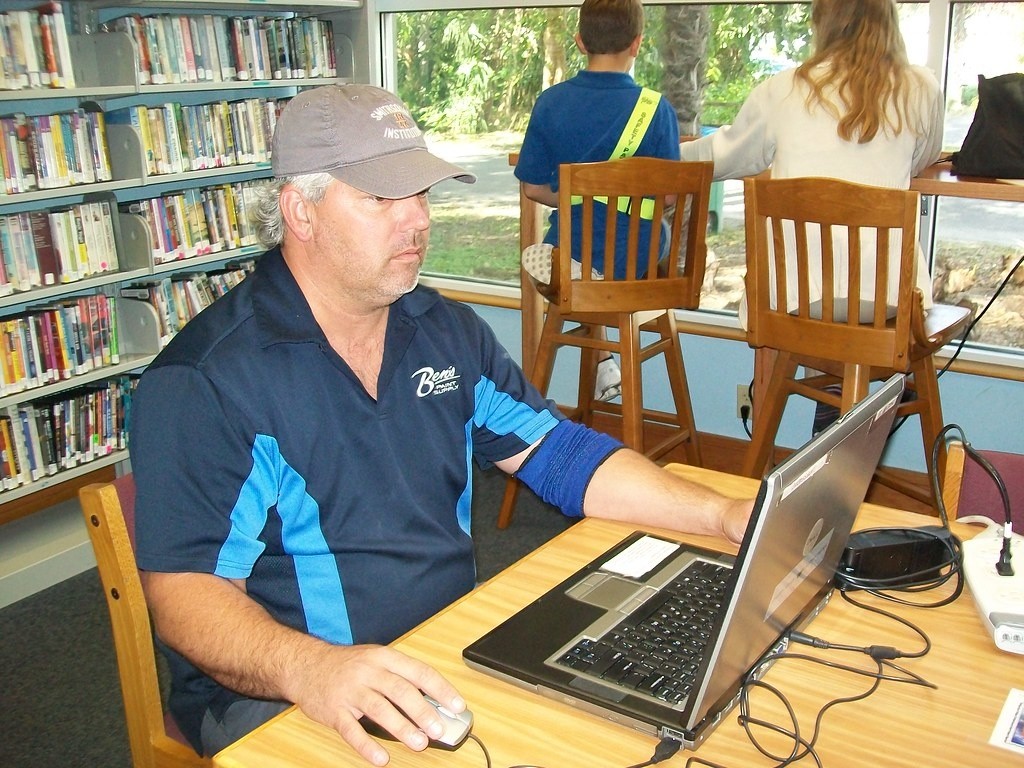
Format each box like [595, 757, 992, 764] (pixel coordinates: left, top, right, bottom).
[959, 522, 1024, 654]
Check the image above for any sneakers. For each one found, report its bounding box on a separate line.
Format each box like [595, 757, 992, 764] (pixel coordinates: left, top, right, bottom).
[585, 358, 630, 405]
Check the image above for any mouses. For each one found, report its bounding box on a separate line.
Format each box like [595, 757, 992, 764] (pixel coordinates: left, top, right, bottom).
[359, 687, 474, 752]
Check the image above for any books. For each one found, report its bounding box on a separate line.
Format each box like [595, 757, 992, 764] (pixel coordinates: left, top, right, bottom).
[2, 3, 338, 509]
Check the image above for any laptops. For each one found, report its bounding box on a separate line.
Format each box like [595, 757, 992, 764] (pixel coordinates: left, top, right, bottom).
[463, 374, 907, 753]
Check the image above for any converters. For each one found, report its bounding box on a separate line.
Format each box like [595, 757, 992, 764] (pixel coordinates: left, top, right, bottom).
[834, 525, 952, 592]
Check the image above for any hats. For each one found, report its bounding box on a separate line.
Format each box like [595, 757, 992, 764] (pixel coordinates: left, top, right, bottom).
[272, 80, 476, 204]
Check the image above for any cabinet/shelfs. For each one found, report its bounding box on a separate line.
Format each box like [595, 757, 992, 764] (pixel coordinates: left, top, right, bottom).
[0, 1, 383, 610]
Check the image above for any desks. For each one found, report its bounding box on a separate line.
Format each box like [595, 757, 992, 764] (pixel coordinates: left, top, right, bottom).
[508, 140, 1024, 449]
[208, 459, 1024, 768]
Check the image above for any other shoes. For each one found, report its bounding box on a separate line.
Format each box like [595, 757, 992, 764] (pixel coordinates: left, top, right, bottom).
[808, 385, 846, 441]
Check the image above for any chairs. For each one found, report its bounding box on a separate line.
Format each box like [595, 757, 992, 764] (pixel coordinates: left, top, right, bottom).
[79, 472, 219, 768]
[745, 177, 976, 508]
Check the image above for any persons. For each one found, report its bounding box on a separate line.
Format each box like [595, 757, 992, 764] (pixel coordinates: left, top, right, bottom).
[125, 85, 760, 766]
[674, 1, 948, 436]
[521, 3, 687, 405]
